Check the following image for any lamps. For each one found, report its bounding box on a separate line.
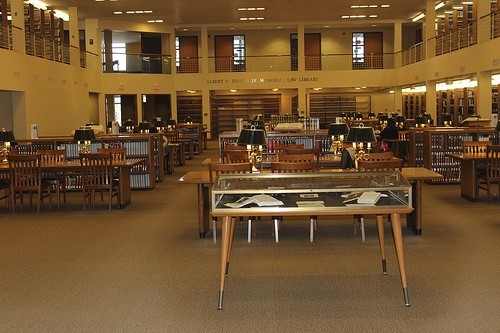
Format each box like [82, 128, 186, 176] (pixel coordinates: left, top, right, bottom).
[243, 121, 267, 137]
[74, 129, 95, 161]
[0, 131, 15, 164]
[238, 129, 265, 171]
[329, 124, 348, 141]
[341, 111, 452, 127]
[347, 128, 377, 170]
[108, 120, 175, 133]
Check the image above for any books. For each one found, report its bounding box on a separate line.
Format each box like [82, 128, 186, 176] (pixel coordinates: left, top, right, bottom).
[342, 191, 382, 204]
[225, 194, 285, 209]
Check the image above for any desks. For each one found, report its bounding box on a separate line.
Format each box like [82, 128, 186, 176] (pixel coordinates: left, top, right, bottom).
[0, 160, 143, 207]
[267, 129, 329, 137]
[178, 168, 444, 238]
[211, 174, 413, 309]
[446, 153, 500, 201]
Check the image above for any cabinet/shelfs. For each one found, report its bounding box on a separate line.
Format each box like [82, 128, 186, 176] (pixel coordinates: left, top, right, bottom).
[18, 135, 154, 190]
[408, 127, 495, 183]
[173, 122, 207, 164]
[155, 138, 175, 182]
[219, 134, 332, 162]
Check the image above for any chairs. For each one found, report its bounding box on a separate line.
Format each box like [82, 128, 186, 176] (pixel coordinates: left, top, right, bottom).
[477, 145, 500, 201]
[208, 144, 403, 241]
[463, 141, 492, 153]
[10, 147, 126, 213]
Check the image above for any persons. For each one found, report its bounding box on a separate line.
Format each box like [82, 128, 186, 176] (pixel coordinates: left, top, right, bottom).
[341, 139, 355, 169]
